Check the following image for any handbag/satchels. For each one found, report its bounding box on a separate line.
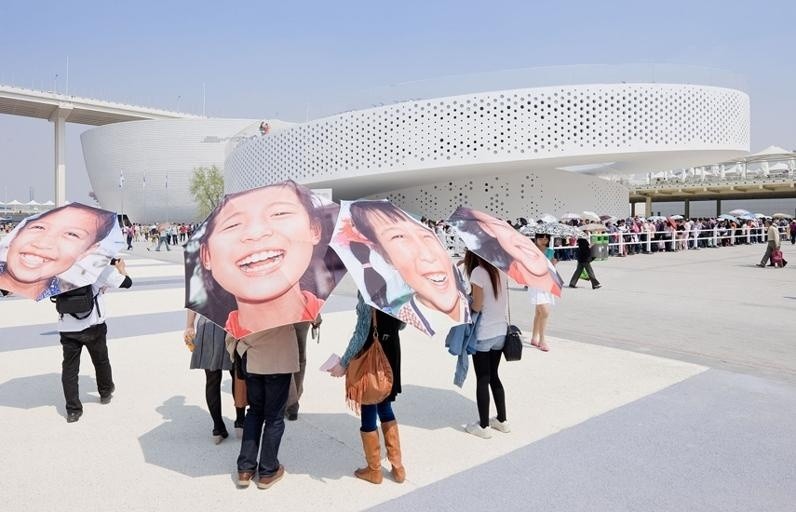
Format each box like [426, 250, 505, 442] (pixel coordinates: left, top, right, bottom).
[658, 240, 665, 249]
[345, 330, 395, 406]
[503, 324, 523, 361]
[579, 269, 590, 280]
[51, 284, 95, 320]
[772, 250, 783, 262]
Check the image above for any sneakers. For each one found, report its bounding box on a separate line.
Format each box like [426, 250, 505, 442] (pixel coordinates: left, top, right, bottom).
[569, 283, 576, 288]
[490, 417, 511, 432]
[592, 285, 601, 289]
[531, 338, 549, 352]
[286, 403, 299, 420]
[67, 409, 82, 423]
[465, 421, 492, 438]
[101, 394, 112, 403]
[756, 263, 787, 268]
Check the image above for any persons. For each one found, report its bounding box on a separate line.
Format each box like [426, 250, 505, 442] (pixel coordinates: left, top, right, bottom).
[568, 230, 602, 290]
[184, 305, 249, 448]
[422, 215, 454, 249]
[50, 255, 131, 428]
[283, 310, 324, 421]
[528, 232, 560, 353]
[348, 197, 472, 336]
[119, 219, 207, 253]
[199, 180, 325, 335]
[758, 220, 787, 267]
[506, 208, 796, 260]
[326, 288, 408, 485]
[0, 218, 19, 235]
[224, 316, 304, 490]
[459, 246, 513, 440]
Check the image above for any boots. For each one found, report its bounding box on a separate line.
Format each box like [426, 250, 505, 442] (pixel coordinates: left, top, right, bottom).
[381, 418, 406, 483]
[355, 427, 381, 484]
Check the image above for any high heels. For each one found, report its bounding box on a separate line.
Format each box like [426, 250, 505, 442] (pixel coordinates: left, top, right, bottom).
[212, 427, 228, 444]
[234, 422, 247, 438]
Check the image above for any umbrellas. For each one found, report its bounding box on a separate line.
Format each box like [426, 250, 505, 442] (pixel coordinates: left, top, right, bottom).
[325, 197, 473, 339]
[180, 177, 349, 340]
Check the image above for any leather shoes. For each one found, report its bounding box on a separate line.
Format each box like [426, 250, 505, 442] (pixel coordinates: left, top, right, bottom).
[238, 471, 256, 486]
[257, 465, 286, 488]
